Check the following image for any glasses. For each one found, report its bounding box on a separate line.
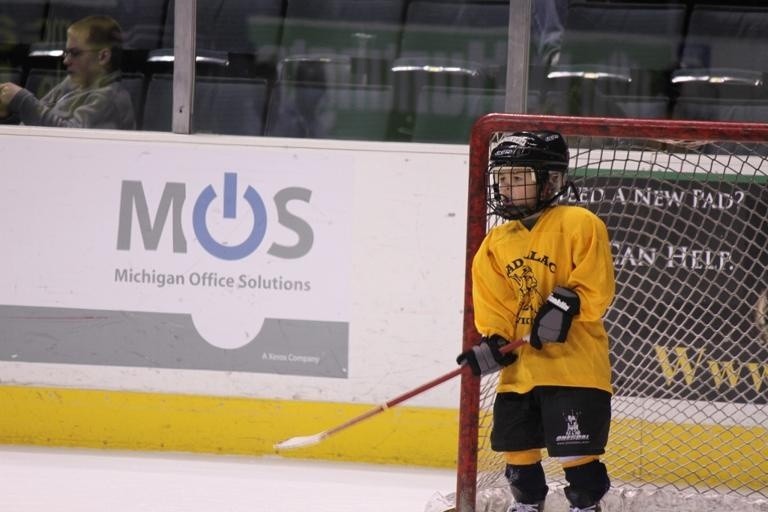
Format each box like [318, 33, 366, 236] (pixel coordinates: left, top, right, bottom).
[62, 47, 99, 57]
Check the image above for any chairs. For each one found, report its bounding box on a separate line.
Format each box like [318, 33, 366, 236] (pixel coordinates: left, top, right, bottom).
[1, 0, 768, 156]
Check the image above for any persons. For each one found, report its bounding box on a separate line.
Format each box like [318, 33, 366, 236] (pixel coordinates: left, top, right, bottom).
[458, 131, 615, 512]
[0, 14, 137, 130]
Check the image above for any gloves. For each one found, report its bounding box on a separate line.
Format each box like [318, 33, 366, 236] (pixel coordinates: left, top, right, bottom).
[528, 287, 580, 350]
[456, 333, 518, 376]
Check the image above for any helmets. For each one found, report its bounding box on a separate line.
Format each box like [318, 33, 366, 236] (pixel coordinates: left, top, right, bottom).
[486, 132, 580, 221]
